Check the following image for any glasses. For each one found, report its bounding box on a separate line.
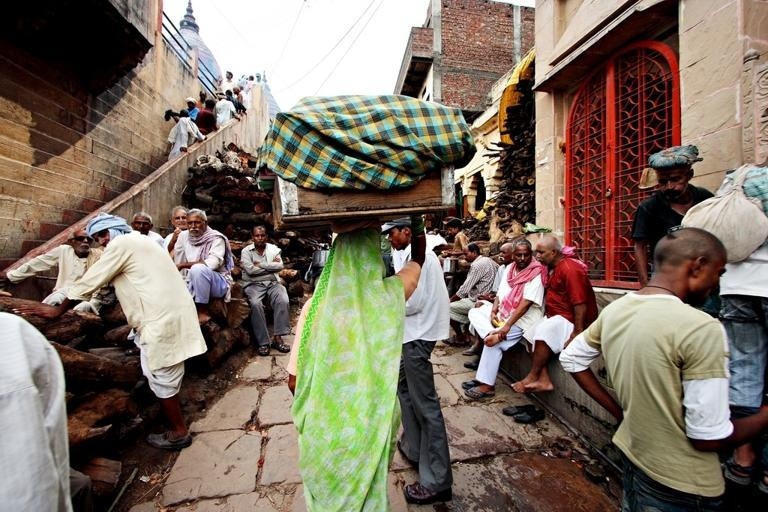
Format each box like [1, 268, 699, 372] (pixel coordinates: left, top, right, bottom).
[70, 235, 91, 241]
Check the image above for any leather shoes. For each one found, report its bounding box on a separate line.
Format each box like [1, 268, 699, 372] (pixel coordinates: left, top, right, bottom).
[503, 403, 546, 423]
[406, 480, 452, 504]
[398, 438, 419, 470]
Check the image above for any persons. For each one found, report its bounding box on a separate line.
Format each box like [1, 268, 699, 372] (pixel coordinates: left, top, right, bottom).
[166, 203, 232, 320]
[3, 211, 164, 312]
[421, 216, 525, 369]
[36, 213, 208, 452]
[381, 218, 453, 503]
[288, 214, 426, 481]
[463, 238, 549, 397]
[164, 72, 264, 158]
[715, 146, 767, 495]
[510, 234, 599, 395]
[559, 228, 768, 511]
[632, 145, 721, 318]
[241, 226, 291, 355]
[0, 311, 73, 512]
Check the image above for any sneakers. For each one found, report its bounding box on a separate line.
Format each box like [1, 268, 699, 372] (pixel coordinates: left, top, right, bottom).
[147, 431, 192, 449]
[258, 345, 270, 356]
[442, 335, 465, 348]
[271, 340, 290, 353]
[462, 346, 496, 398]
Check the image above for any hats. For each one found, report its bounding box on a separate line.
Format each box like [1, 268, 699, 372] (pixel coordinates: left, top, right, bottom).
[381, 217, 412, 234]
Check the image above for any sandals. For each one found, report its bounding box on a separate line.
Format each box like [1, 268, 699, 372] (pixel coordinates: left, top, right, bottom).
[724, 455, 768, 494]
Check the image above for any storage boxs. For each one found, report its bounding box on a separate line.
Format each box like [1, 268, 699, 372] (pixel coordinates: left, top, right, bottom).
[271, 161, 442, 233]
